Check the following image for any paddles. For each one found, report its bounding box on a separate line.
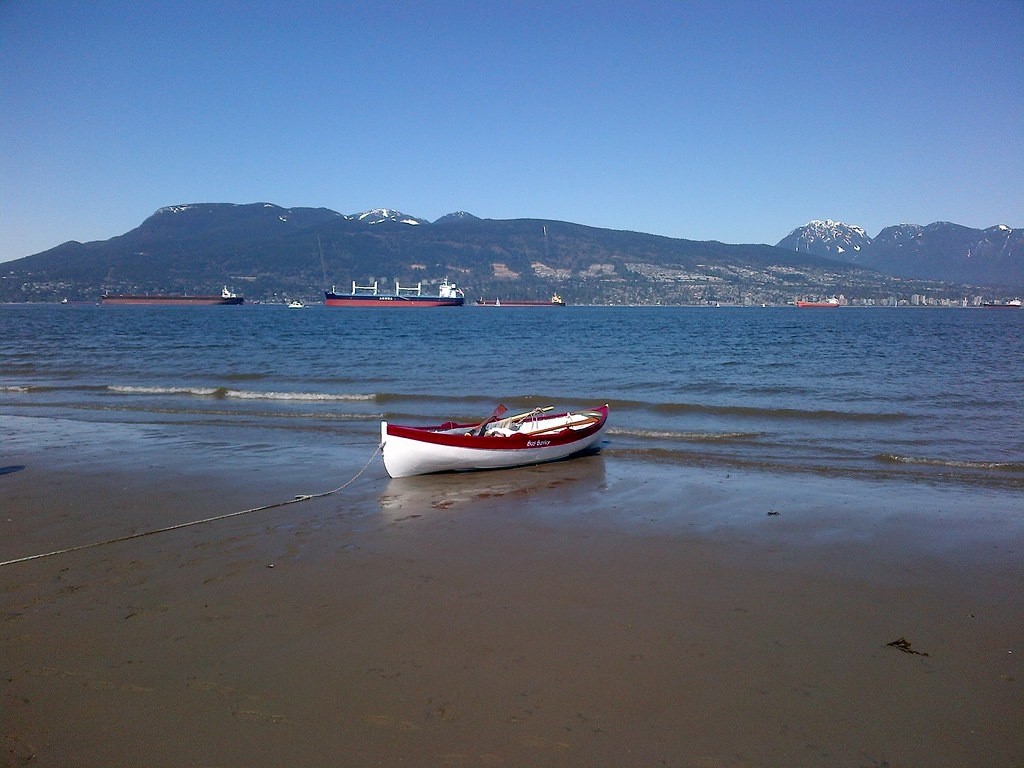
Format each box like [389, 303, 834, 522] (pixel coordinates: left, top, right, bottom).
[525, 417, 599, 436]
[464, 403, 508, 436]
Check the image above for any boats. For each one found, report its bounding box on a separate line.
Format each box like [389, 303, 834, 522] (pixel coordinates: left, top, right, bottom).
[381, 404, 609, 480]
[100, 285, 244, 305]
[796, 295, 840, 307]
[980, 297, 1022, 307]
[324, 279, 465, 307]
[287, 300, 309, 309]
[475, 292, 566, 307]
